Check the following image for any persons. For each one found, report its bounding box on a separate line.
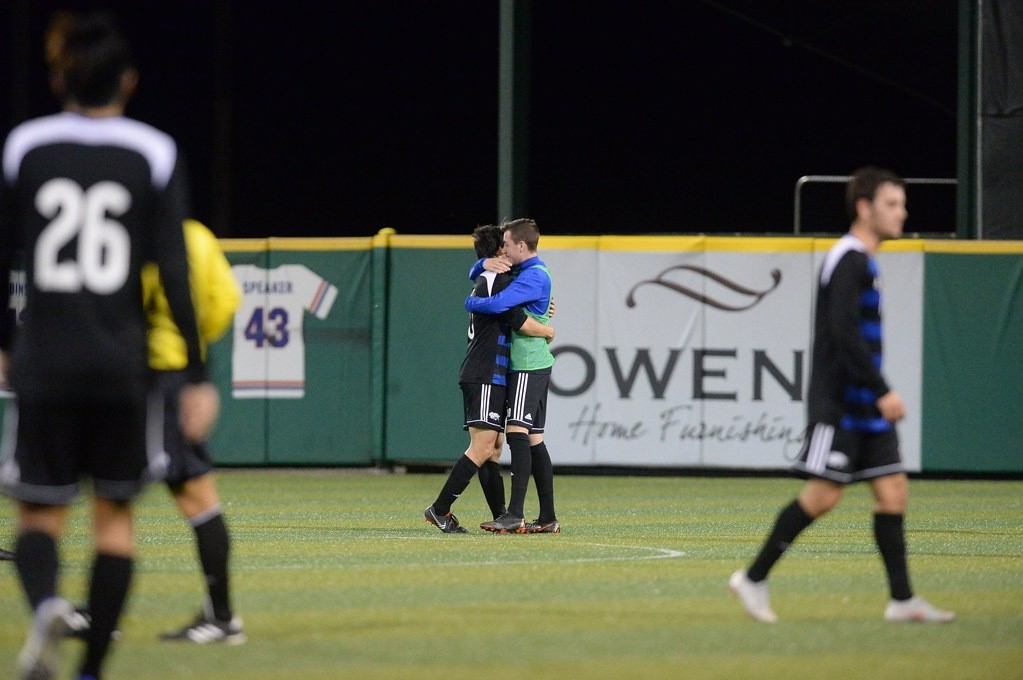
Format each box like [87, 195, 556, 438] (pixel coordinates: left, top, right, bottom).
[723, 166, 959, 625]
[422, 216, 562, 535]
[52, 164, 248, 651]
[1, 11, 209, 680]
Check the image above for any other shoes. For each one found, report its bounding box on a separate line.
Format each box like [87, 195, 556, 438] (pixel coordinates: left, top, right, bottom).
[19, 597, 72, 680]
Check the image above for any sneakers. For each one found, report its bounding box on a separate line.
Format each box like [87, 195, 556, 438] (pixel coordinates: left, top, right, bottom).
[885, 597, 957, 625]
[425, 507, 467, 533]
[522, 520, 560, 534]
[481, 513, 525, 534]
[729, 570, 778, 623]
[159, 616, 243, 647]
[58, 607, 119, 640]
